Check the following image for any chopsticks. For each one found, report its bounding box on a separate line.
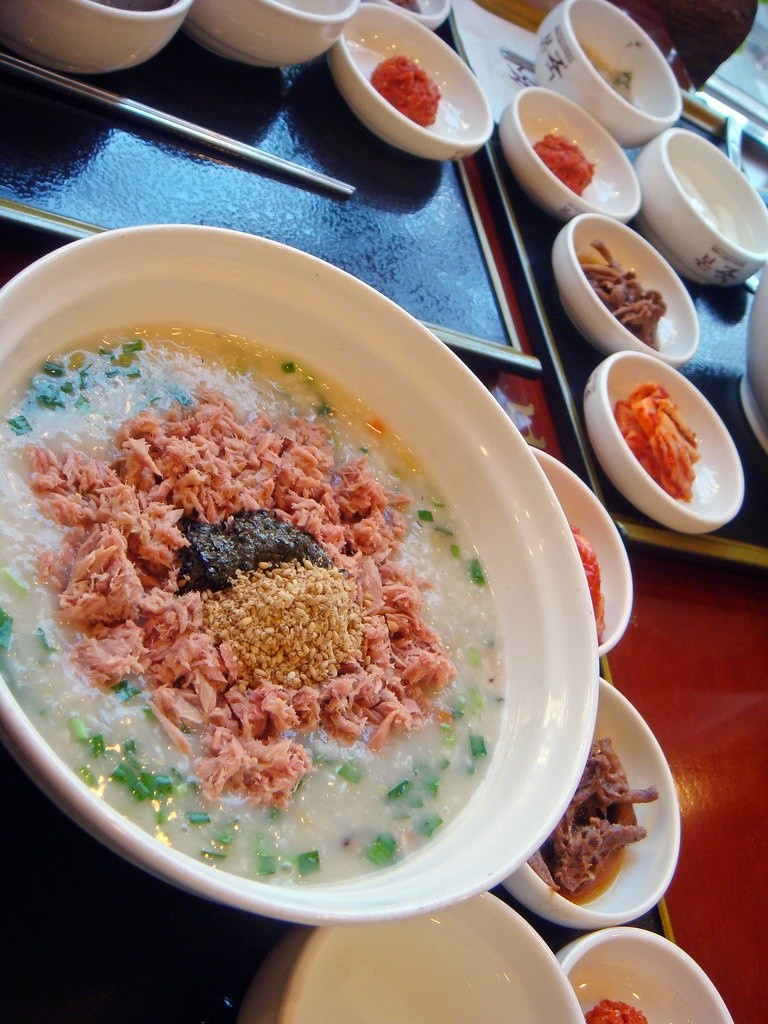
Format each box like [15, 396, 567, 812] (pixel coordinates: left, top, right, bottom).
[0, 51, 357, 198]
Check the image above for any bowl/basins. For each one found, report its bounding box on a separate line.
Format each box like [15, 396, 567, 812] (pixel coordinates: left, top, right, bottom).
[0, 221, 600, 926]
[744, 258, 768, 436]
[327, 2, 494, 161]
[631, 128, 768, 288]
[359, 0, 451, 30]
[582, 350, 746, 534]
[180, 0, 361, 72]
[498, 675, 681, 931]
[532, 0, 684, 149]
[524, 445, 634, 659]
[551, 212, 700, 370]
[0, 0, 194, 74]
[230, 888, 587, 1024]
[552, 926, 734, 1024]
[499, 84, 641, 226]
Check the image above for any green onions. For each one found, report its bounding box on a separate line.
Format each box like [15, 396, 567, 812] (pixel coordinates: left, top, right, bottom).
[0, 342, 491, 876]
